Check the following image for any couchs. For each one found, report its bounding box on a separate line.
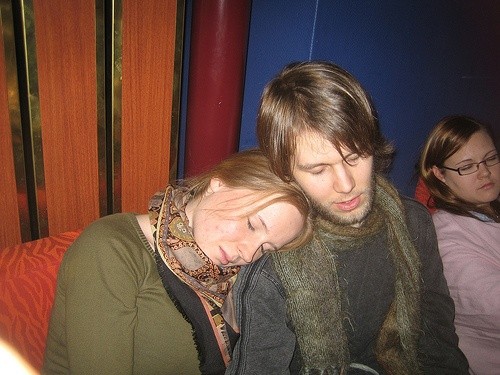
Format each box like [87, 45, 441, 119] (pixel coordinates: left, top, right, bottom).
[0, 231, 79, 374]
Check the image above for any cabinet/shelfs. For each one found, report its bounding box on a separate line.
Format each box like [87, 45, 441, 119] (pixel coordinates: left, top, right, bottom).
[1, 1, 186, 248]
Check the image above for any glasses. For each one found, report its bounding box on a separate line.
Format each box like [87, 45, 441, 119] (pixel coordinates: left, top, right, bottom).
[442, 153, 500, 176]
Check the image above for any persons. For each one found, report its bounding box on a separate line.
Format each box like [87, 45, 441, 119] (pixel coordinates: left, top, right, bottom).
[39, 146, 315, 375]
[223, 60, 470, 375]
[417, 114, 500, 375]
